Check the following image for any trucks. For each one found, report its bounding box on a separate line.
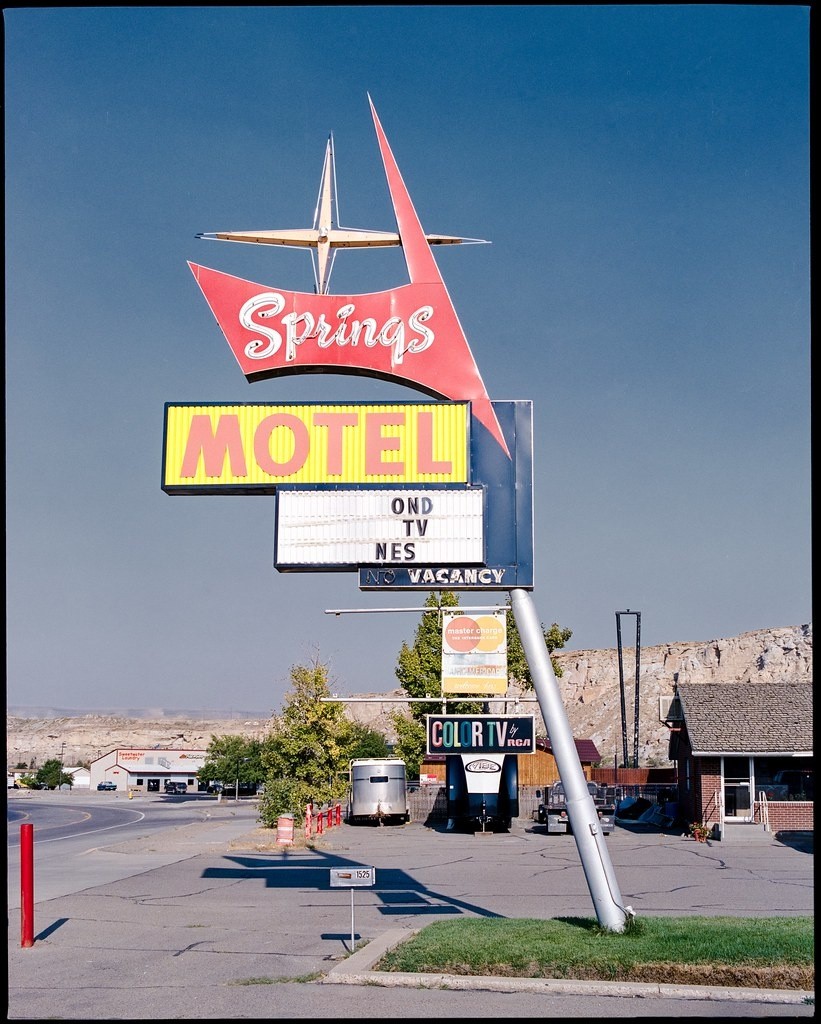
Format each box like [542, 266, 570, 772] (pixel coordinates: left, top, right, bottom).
[547, 779, 615, 833]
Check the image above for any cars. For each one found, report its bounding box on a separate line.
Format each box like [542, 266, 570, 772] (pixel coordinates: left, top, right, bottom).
[206, 784, 226, 795]
[97, 781, 117, 791]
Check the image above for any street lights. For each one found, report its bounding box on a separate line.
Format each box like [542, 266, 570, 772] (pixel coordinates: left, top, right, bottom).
[235, 758, 248, 800]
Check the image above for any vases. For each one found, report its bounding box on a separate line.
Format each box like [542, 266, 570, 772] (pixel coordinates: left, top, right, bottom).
[695, 830, 707, 841]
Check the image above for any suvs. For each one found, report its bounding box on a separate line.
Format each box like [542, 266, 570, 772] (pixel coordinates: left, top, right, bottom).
[164, 782, 189, 795]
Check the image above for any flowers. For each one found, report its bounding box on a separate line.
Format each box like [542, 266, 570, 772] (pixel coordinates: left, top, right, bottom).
[689, 822, 709, 840]
